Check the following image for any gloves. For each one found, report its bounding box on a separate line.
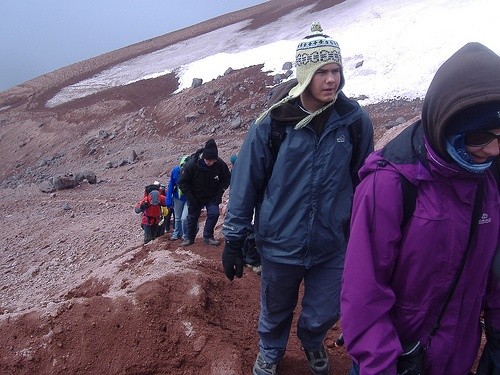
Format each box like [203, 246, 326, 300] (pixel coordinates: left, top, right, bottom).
[221, 242, 243, 281]
[141, 201, 150, 209]
[476, 344, 499, 375]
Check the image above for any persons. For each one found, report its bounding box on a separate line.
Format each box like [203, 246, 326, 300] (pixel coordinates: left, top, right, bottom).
[134, 153, 190, 244]
[338, 42, 500, 375]
[222, 33, 375, 375]
[178, 143, 231, 245]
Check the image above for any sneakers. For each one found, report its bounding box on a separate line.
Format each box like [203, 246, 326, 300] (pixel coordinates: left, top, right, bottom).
[252, 352, 279, 375]
[304, 343, 330, 375]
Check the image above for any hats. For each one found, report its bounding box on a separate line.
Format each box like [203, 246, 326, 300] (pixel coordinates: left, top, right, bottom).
[154, 180, 160, 186]
[448, 103, 500, 150]
[203, 138, 218, 160]
[255, 21, 346, 130]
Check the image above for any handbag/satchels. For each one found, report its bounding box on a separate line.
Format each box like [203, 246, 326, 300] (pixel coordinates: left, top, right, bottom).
[399, 339, 425, 375]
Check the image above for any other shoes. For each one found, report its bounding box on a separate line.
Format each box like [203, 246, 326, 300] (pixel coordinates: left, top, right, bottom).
[203, 237, 220, 246]
[181, 239, 194, 246]
[246, 263, 262, 274]
[170, 234, 181, 240]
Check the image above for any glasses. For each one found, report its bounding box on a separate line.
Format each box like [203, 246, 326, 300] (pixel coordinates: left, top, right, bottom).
[466, 130, 500, 147]
